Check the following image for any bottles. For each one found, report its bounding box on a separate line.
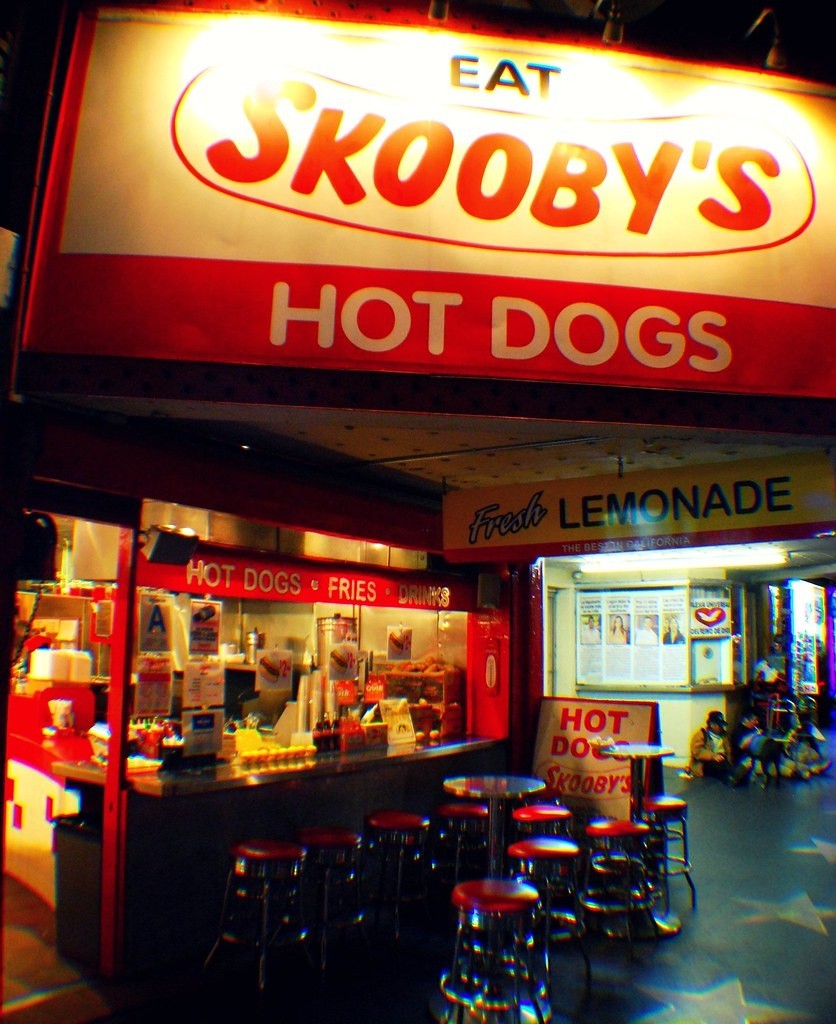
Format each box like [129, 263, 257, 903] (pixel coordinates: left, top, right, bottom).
[317, 710, 339, 753]
[127, 715, 168, 746]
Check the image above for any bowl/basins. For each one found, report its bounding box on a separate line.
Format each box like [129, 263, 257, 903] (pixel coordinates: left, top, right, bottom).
[592, 744, 612, 751]
[225, 653, 246, 664]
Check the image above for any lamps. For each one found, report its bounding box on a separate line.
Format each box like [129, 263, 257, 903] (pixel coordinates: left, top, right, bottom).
[746, 6, 786, 69]
[588, 0, 623, 45]
[137, 525, 198, 565]
[427, 0, 448, 21]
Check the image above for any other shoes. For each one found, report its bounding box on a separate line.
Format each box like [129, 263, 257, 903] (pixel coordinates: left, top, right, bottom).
[809, 760, 830, 774]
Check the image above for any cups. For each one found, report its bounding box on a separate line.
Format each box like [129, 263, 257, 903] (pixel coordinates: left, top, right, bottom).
[297, 669, 337, 733]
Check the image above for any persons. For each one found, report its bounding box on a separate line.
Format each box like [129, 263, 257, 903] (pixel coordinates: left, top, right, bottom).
[736, 709, 831, 779]
[582, 617, 600, 644]
[689, 711, 749, 786]
[607, 615, 628, 644]
[635, 616, 658, 646]
[663, 615, 685, 643]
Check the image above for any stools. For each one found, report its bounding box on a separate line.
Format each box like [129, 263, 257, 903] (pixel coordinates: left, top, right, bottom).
[205, 797, 697, 1024]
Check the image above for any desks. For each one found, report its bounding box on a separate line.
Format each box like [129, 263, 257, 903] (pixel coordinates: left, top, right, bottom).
[607, 747, 676, 822]
[440, 773, 546, 881]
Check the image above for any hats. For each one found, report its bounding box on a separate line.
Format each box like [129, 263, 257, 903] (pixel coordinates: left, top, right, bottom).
[709, 711, 728, 726]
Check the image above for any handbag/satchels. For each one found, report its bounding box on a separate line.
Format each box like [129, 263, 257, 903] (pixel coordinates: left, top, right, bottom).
[750, 734, 781, 790]
